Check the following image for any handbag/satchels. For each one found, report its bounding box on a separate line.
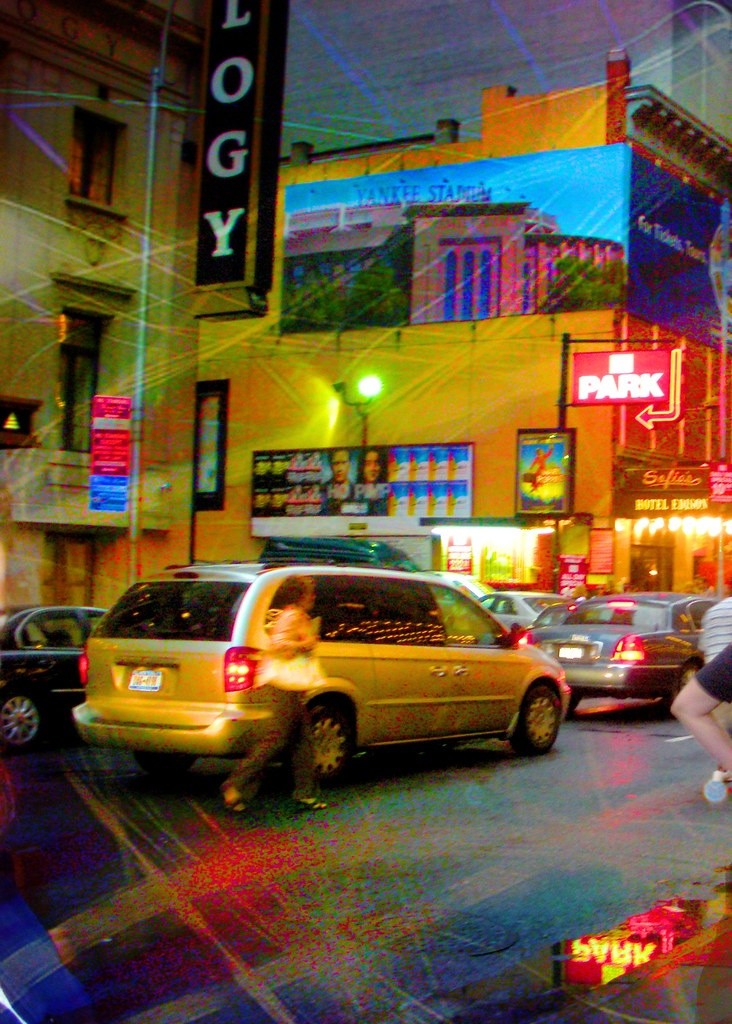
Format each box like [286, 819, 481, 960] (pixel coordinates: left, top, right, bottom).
[257, 611, 325, 691]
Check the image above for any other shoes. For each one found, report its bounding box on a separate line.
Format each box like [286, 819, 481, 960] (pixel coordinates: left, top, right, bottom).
[712, 770, 732, 783]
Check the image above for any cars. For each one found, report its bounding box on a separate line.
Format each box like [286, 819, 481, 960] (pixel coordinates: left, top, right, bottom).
[1, 606, 110, 752]
[256, 535, 721, 719]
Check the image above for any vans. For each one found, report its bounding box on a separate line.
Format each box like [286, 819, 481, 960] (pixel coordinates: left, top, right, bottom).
[71, 558, 574, 784]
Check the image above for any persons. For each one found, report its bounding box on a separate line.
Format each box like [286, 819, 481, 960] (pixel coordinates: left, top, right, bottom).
[532, 445, 554, 490]
[328, 450, 393, 504]
[216, 577, 329, 818]
[673, 591, 732, 782]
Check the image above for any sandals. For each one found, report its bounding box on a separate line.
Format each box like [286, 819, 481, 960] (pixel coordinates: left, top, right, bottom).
[223, 786, 247, 812]
[294, 799, 328, 810]
